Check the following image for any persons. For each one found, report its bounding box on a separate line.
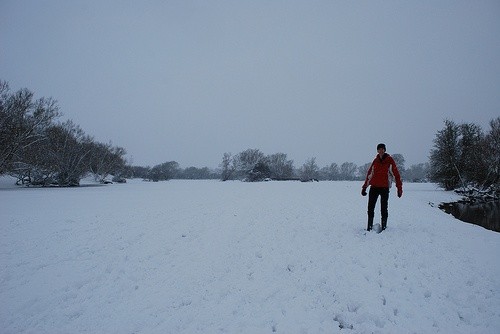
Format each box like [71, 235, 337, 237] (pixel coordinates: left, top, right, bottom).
[360, 143, 403, 233]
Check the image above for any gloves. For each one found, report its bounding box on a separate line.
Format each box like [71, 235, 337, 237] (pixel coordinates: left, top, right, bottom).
[361, 188, 367, 196]
[397, 191, 402, 198]
